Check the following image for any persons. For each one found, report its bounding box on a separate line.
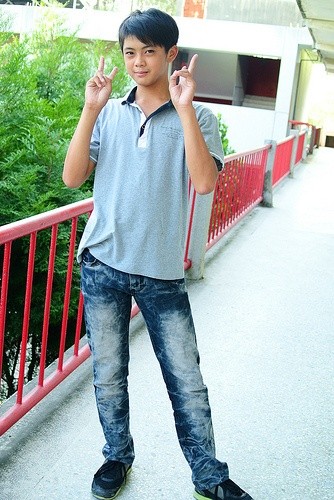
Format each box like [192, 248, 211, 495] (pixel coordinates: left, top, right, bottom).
[62, 7, 254, 500]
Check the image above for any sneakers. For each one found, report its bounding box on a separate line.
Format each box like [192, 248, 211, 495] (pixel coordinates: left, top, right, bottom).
[92, 459, 132, 500]
[193, 479, 254, 500]
